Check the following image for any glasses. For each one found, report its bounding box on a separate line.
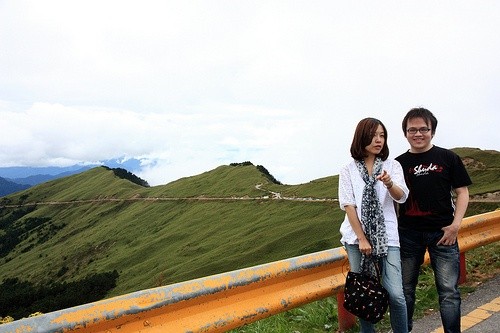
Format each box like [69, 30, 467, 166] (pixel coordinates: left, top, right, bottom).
[405, 126, 433, 134]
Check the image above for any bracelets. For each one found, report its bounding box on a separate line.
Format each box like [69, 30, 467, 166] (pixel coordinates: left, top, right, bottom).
[387, 181, 393, 189]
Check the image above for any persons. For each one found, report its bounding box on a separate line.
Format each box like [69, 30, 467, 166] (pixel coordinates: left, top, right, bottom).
[339, 118, 409, 333]
[393, 108, 473, 333]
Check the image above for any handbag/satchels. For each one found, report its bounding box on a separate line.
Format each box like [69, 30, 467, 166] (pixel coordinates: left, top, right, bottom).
[342, 252, 390, 324]
